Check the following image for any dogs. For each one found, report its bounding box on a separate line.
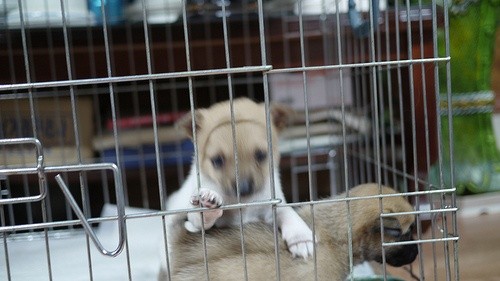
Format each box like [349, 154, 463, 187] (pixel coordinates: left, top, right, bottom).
[165, 98, 318, 261]
[156, 182, 419, 281]
